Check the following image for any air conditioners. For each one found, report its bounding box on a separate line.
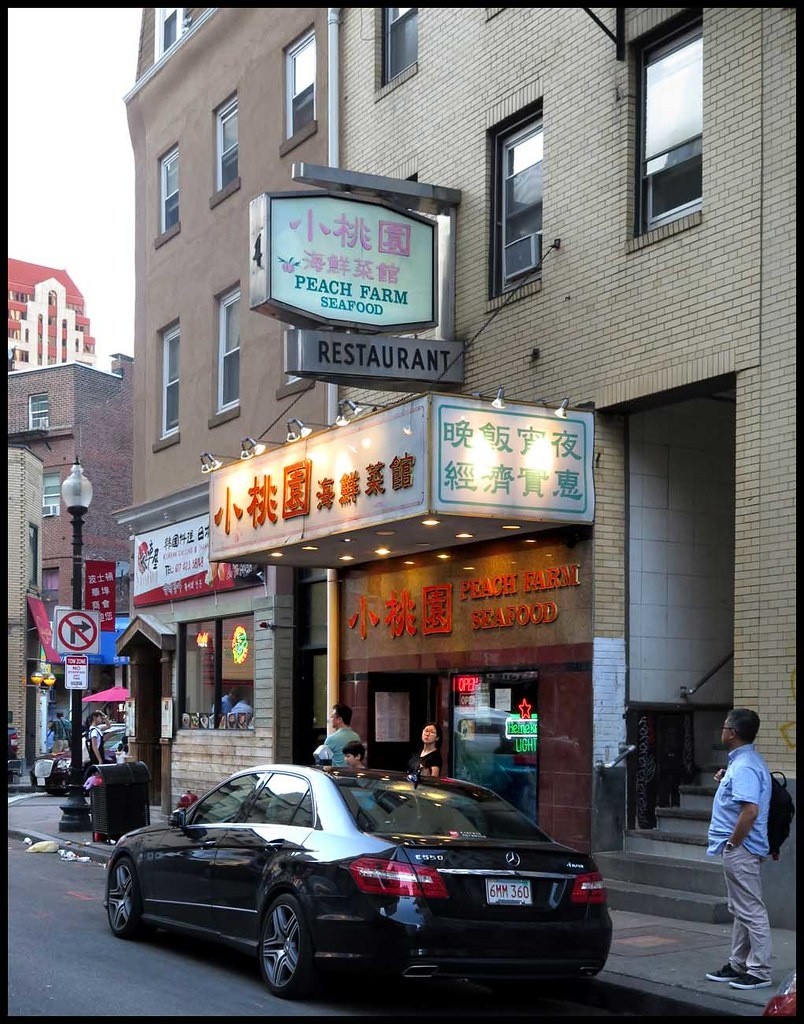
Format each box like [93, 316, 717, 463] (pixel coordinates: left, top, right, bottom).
[32, 417, 46, 429]
[19, 671, 45, 688]
[39, 673, 56, 688]
[43, 504, 58, 517]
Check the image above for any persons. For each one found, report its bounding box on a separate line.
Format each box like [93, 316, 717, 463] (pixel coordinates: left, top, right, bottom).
[121, 736, 129, 754]
[117, 744, 126, 764]
[51, 712, 72, 753]
[46, 721, 55, 754]
[212, 689, 235, 716]
[85, 710, 111, 766]
[343, 741, 365, 768]
[410, 723, 442, 777]
[707, 709, 773, 988]
[231, 688, 253, 717]
[323, 704, 361, 767]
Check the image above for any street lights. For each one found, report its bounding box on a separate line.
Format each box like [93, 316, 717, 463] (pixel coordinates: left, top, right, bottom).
[59, 457, 92, 832]
[30, 670, 56, 757]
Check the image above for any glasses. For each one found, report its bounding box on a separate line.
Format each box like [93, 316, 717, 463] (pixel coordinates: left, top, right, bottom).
[718, 725, 738, 735]
[423, 730, 436, 736]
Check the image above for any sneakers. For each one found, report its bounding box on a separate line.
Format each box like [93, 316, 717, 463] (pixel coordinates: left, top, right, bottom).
[705, 964, 737, 983]
[730, 972, 773, 991]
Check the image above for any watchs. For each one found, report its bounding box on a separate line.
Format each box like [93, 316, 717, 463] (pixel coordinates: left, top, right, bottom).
[726, 842, 735, 850]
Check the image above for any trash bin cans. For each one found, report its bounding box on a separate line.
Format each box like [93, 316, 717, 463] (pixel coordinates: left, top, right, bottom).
[83, 761, 150, 846]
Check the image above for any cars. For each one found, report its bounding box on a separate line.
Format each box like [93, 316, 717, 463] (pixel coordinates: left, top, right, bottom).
[493, 721, 538, 789]
[104, 764, 612, 998]
[29, 724, 126, 795]
[454, 706, 511, 781]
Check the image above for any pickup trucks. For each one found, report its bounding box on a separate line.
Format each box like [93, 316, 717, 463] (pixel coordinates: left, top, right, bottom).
[8, 711, 21, 772]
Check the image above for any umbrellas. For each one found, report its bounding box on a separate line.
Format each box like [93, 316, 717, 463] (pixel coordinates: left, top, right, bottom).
[82, 686, 129, 702]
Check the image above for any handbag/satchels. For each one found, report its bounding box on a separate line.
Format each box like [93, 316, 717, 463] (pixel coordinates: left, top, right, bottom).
[766, 768, 794, 860]
[67, 737, 72, 750]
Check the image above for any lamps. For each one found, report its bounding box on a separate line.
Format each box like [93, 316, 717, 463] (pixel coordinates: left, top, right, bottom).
[285, 418, 334, 442]
[238, 436, 283, 460]
[472, 385, 508, 408]
[543, 396, 570, 419]
[333, 396, 386, 428]
[199, 450, 241, 473]
